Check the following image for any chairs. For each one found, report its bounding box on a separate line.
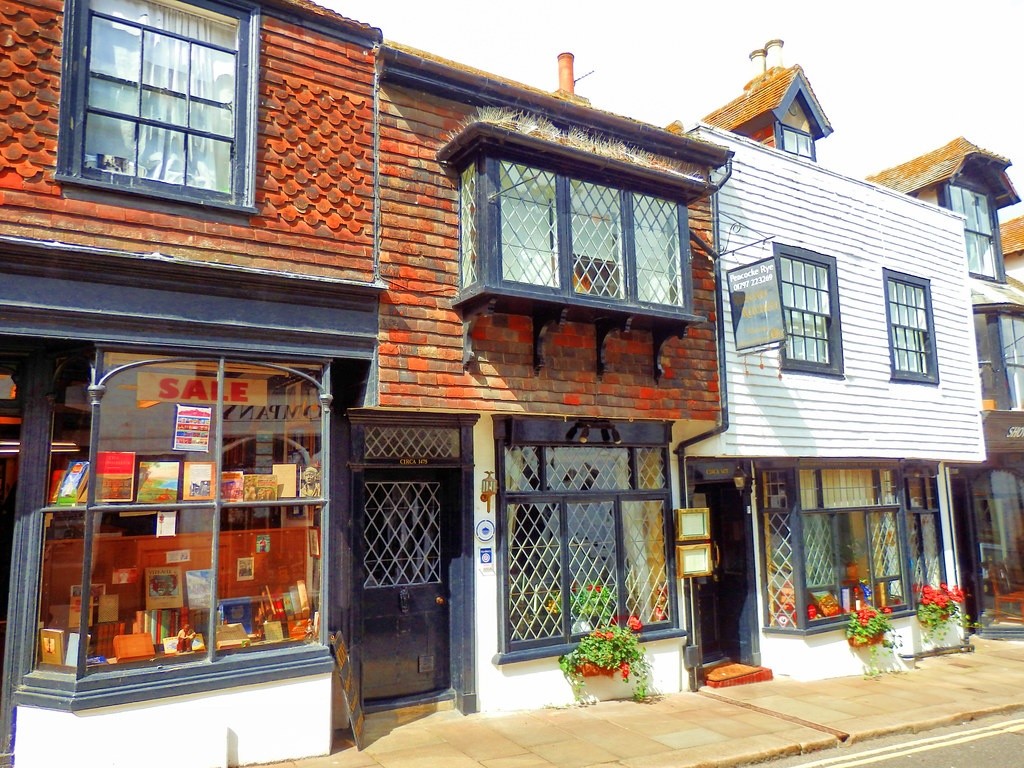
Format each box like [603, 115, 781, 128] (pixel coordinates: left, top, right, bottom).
[986, 559, 1024, 625]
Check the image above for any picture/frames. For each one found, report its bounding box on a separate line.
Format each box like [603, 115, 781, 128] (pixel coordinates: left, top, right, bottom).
[52, 451, 216, 507]
[309, 525, 320, 559]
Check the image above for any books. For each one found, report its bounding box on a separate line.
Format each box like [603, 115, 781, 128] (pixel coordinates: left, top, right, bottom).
[35, 561, 313, 664]
[46, 450, 323, 506]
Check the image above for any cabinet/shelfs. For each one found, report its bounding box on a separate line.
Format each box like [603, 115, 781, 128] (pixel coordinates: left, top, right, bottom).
[36, 495, 326, 667]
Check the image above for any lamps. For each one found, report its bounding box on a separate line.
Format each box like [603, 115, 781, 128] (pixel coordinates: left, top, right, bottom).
[575, 421, 622, 444]
[733, 465, 748, 495]
[481, 471, 497, 513]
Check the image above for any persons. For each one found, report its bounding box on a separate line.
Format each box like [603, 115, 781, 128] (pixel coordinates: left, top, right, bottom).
[177, 623, 196, 653]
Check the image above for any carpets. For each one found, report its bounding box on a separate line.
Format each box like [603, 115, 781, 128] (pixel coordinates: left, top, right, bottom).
[702, 661, 762, 681]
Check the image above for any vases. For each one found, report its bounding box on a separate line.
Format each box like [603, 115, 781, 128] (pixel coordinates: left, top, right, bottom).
[921, 613, 949, 626]
[577, 659, 624, 677]
[849, 634, 883, 648]
[570, 617, 591, 633]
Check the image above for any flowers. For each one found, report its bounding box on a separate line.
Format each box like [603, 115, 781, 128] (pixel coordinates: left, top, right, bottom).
[841, 605, 901, 679]
[547, 582, 654, 705]
[916, 584, 981, 647]
